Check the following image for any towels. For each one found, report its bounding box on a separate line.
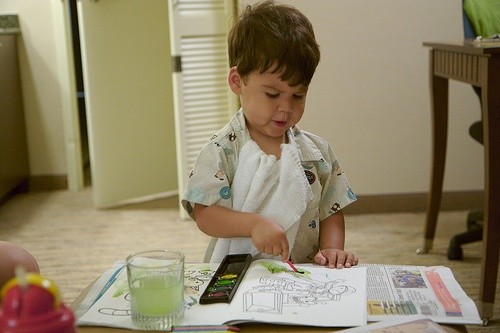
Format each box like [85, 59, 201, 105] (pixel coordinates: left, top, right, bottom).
[208, 139, 315, 263]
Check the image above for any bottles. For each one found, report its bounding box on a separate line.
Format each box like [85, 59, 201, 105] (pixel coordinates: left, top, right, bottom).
[0, 263, 77, 333]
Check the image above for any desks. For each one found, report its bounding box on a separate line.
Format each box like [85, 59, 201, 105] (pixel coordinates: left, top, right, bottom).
[419, 42, 500, 320]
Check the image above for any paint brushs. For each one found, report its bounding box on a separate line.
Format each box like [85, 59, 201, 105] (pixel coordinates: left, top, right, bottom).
[283, 257, 307, 276]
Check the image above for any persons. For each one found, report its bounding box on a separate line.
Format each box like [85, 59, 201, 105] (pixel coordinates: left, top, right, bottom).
[181, 1, 359, 269]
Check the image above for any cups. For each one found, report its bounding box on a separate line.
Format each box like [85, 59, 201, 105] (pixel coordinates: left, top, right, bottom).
[125, 249, 186, 330]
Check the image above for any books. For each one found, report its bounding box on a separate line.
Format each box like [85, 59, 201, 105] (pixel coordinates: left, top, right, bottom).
[75, 258, 368, 331]
